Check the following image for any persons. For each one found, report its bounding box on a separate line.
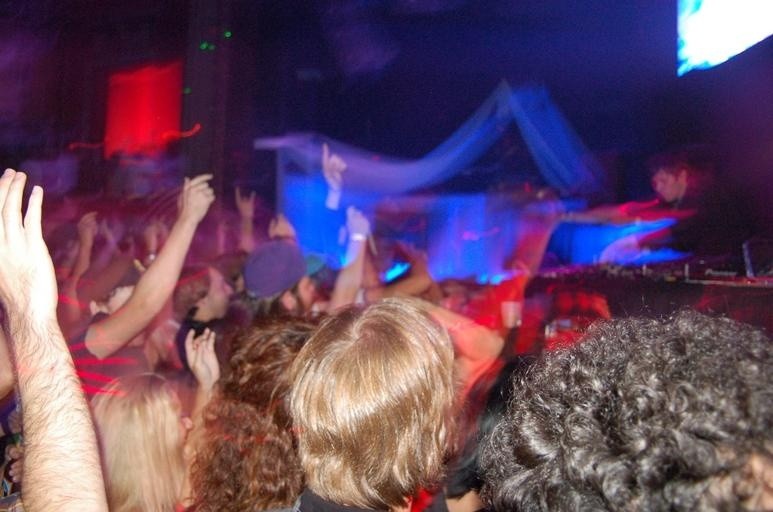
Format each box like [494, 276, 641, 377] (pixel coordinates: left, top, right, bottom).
[1, 138, 772, 512]
